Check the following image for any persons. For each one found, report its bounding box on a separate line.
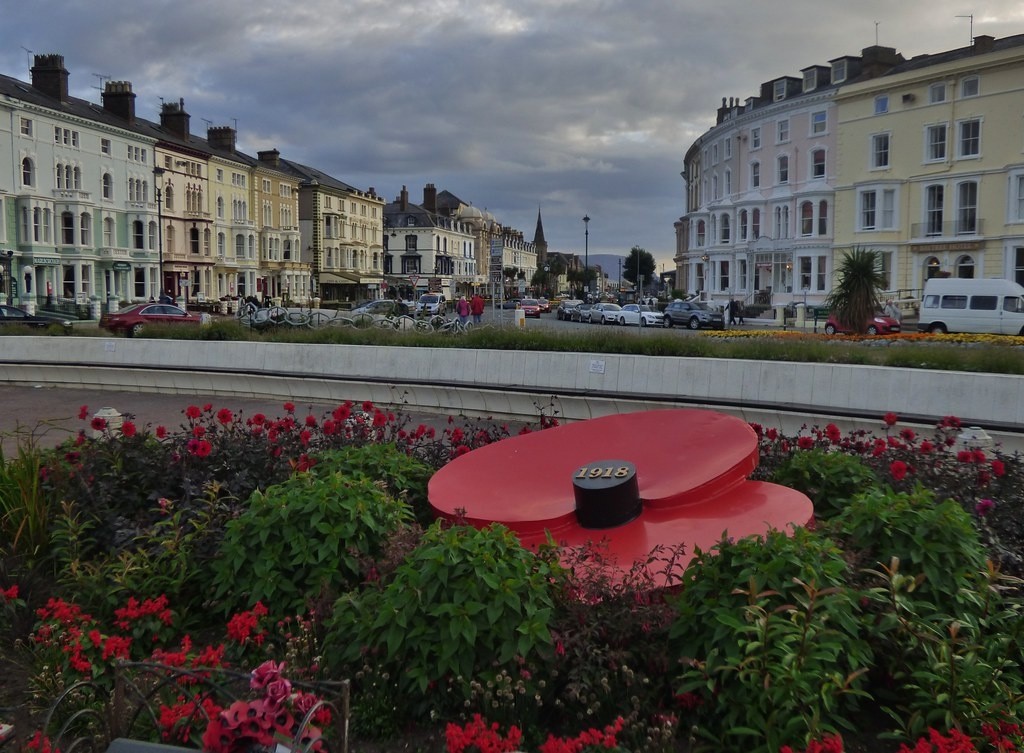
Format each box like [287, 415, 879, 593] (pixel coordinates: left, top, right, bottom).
[737, 302, 745, 325]
[394, 298, 410, 317]
[725, 297, 738, 326]
[884, 299, 902, 321]
[456, 295, 469, 324]
[471, 294, 484, 324]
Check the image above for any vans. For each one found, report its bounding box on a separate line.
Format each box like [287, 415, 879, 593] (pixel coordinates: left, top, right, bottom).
[917, 278, 1024, 337]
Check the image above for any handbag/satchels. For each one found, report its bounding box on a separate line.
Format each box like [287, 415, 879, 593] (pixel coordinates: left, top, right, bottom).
[457, 307, 461, 314]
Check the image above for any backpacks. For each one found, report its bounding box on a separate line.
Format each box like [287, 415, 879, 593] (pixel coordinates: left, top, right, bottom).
[397, 303, 409, 315]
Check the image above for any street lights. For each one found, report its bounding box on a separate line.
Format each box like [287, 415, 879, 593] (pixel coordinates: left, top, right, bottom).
[153, 165, 173, 305]
[618, 258, 622, 305]
[582, 213, 590, 295]
[543, 262, 552, 298]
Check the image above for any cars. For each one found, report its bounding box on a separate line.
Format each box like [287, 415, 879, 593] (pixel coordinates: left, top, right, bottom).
[520, 298, 541, 318]
[825, 306, 903, 337]
[0, 304, 74, 337]
[496, 298, 520, 309]
[98, 303, 200, 337]
[558, 299, 584, 321]
[571, 303, 593, 324]
[414, 293, 447, 316]
[588, 302, 622, 325]
[618, 303, 670, 329]
[665, 301, 725, 331]
[346, 299, 408, 322]
[537, 298, 551, 314]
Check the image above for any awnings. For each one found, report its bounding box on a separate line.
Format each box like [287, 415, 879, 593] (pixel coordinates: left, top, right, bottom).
[319, 272, 384, 284]
